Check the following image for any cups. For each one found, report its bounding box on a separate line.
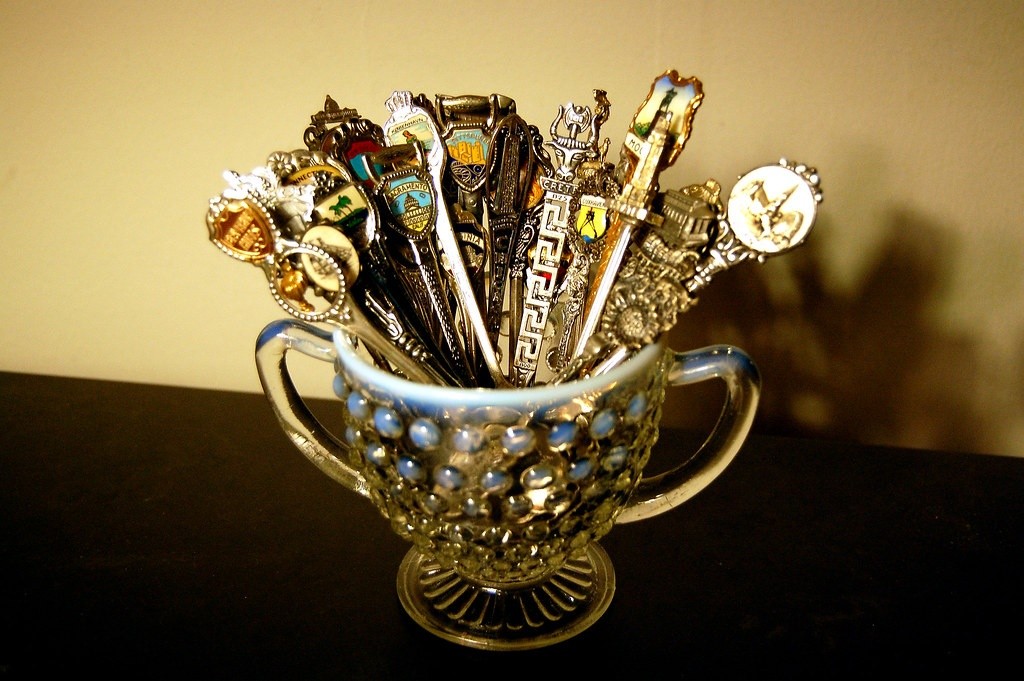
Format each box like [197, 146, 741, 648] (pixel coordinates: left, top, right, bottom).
[253, 320, 758, 650]
